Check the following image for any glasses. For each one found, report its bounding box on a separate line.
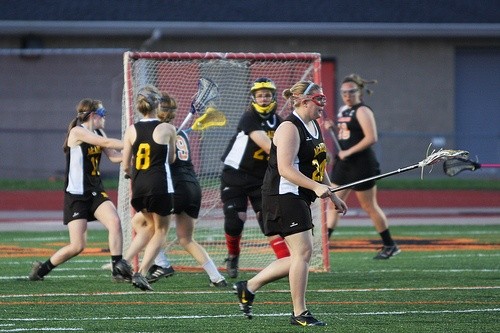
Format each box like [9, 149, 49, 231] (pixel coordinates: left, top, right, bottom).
[340, 88, 360, 95]
[301, 94, 327, 106]
[78, 107, 107, 117]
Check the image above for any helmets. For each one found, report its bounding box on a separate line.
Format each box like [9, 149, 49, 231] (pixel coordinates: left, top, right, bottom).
[247, 77, 278, 118]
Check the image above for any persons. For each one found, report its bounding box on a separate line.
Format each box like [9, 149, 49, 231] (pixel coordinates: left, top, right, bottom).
[29, 98, 137, 282]
[234, 81, 348, 326]
[324, 75, 402, 261]
[218, 78, 290, 278]
[124, 86, 228, 290]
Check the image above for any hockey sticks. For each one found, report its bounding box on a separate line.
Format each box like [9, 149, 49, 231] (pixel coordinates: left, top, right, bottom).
[444, 158, 500, 177]
[184, 106, 227, 136]
[176, 77, 218, 136]
[323, 148, 469, 192]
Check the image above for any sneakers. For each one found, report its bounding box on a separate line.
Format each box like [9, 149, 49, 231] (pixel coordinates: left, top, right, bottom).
[231, 279, 256, 320]
[223, 252, 240, 278]
[131, 272, 154, 291]
[372, 242, 402, 259]
[110, 272, 131, 284]
[144, 264, 174, 283]
[289, 309, 328, 326]
[115, 260, 134, 280]
[28, 260, 44, 281]
[206, 275, 227, 287]
